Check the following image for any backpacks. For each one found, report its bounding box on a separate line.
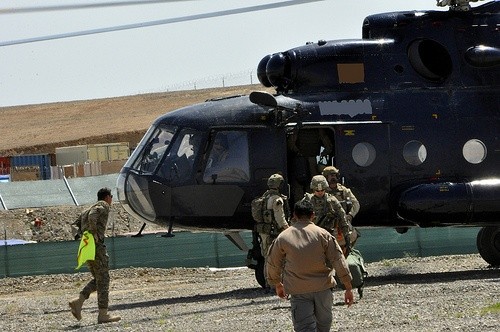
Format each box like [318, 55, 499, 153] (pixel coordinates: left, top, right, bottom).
[252, 190, 280, 234]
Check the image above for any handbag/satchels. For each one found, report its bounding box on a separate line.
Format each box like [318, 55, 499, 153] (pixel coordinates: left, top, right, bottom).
[336, 248, 365, 291]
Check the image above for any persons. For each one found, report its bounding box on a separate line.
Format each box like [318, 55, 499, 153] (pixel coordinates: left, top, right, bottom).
[204, 132, 231, 184]
[258, 174, 289, 295]
[321, 166, 360, 258]
[268, 201, 354, 332]
[69, 188, 121, 323]
[301, 174, 351, 252]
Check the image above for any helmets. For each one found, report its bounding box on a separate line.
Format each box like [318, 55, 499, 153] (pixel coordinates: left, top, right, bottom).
[320, 167, 341, 177]
[268, 174, 286, 192]
[310, 175, 331, 190]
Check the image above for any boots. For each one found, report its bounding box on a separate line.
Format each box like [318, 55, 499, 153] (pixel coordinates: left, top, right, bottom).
[69, 291, 85, 322]
[97, 308, 118, 322]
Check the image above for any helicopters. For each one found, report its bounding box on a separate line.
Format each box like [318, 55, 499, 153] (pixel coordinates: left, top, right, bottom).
[0, 0, 500, 291]
